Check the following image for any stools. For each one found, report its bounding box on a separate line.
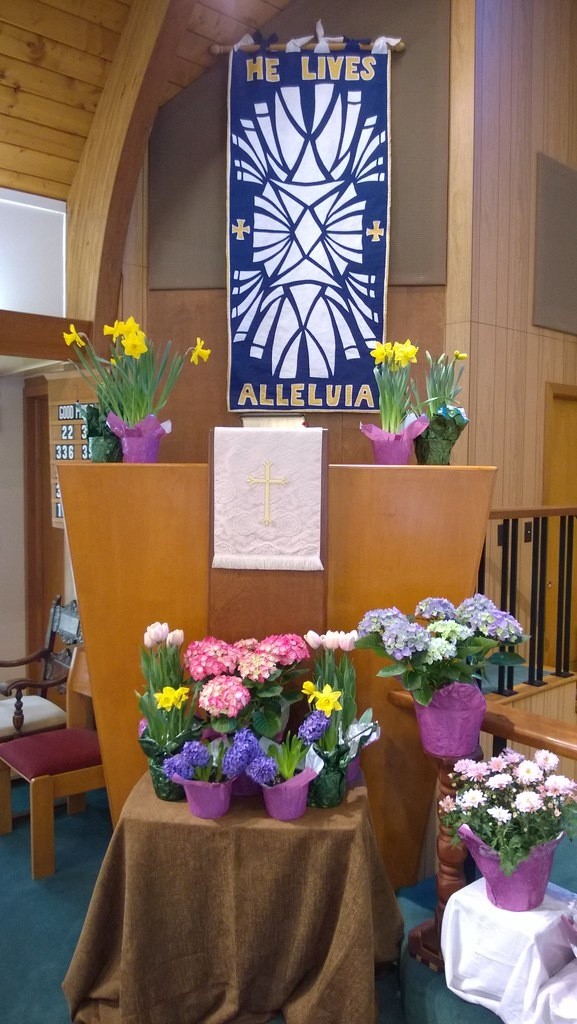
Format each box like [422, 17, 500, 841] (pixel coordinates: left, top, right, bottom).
[440, 876, 577, 1024]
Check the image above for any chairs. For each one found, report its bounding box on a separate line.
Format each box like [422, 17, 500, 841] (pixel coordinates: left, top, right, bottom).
[0, 595, 85, 744]
[0, 647, 107, 880]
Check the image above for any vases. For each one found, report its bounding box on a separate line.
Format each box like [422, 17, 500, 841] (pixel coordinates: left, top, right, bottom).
[74, 402, 122, 464]
[409, 684, 487, 756]
[358, 415, 430, 465]
[139, 724, 211, 801]
[459, 824, 568, 911]
[106, 413, 172, 464]
[171, 734, 241, 818]
[410, 407, 470, 465]
[296, 724, 376, 809]
[255, 737, 325, 821]
[347, 726, 384, 782]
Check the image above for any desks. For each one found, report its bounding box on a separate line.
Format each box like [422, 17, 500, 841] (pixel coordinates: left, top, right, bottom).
[60, 771, 405, 1024]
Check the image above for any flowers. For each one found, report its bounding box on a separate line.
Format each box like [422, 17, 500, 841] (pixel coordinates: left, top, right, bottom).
[370, 338, 462, 432]
[63, 316, 212, 431]
[355, 594, 530, 708]
[404, 350, 468, 418]
[436, 746, 577, 879]
[133, 620, 358, 785]
